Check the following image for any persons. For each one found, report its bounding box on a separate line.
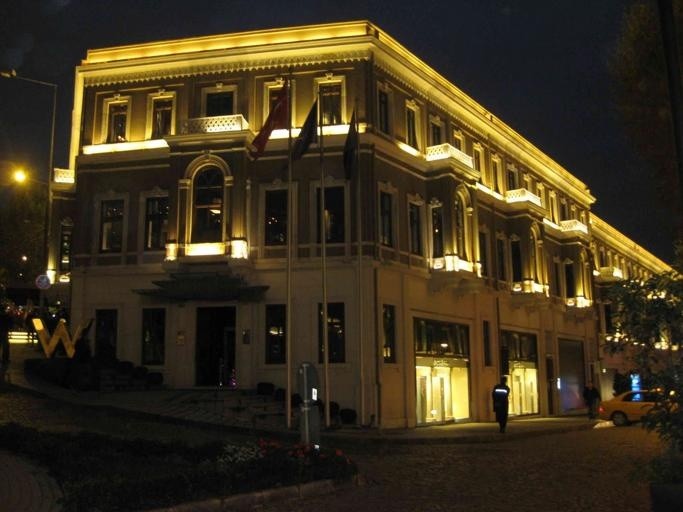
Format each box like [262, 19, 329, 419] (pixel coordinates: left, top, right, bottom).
[582, 380, 601, 420]
[490, 376, 510, 432]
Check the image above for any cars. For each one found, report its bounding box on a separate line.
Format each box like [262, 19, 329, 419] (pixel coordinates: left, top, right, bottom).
[598, 389, 678, 426]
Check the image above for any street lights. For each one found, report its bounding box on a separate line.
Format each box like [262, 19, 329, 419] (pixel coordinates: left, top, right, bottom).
[0, 71, 58, 352]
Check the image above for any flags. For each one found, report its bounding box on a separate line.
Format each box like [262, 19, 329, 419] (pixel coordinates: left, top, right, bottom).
[342, 109, 359, 181]
[292, 98, 318, 162]
[249, 79, 289, 154]
